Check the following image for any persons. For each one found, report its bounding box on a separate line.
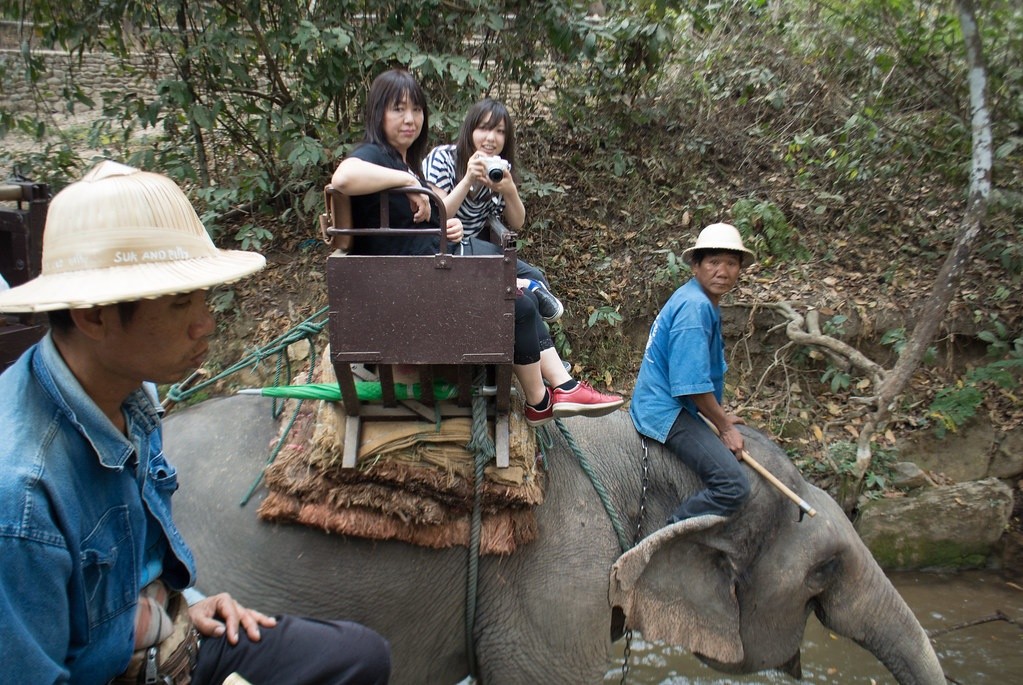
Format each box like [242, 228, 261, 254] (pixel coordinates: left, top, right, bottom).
[0, 160, 390, 685]
[422, 98, 571, 373]
[331, 70, 624, 425]
[630, 221, 756, 525]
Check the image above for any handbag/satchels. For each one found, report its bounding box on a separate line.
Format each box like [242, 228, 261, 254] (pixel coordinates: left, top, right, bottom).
[116, 587, 202, 685]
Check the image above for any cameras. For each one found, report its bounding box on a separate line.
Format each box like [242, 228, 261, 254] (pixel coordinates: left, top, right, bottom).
[478, 155, 511, 182]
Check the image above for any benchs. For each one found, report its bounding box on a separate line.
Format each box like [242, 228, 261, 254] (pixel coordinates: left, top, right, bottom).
[316, 184, 519, 472]
[0, 181, 53, 376]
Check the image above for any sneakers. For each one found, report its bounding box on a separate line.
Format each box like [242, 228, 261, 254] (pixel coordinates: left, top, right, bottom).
[524, 385, 553, 427]
[552, 379, 625, 418]
[531, 278, 564, 323]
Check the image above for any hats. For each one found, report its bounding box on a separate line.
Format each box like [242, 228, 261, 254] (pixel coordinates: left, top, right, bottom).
[0, 159, 266, 313]
[682, 223, 757, 269]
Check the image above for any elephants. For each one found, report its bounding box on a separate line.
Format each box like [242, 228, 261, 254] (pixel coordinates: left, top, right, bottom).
[158, 392, 946, 685]
